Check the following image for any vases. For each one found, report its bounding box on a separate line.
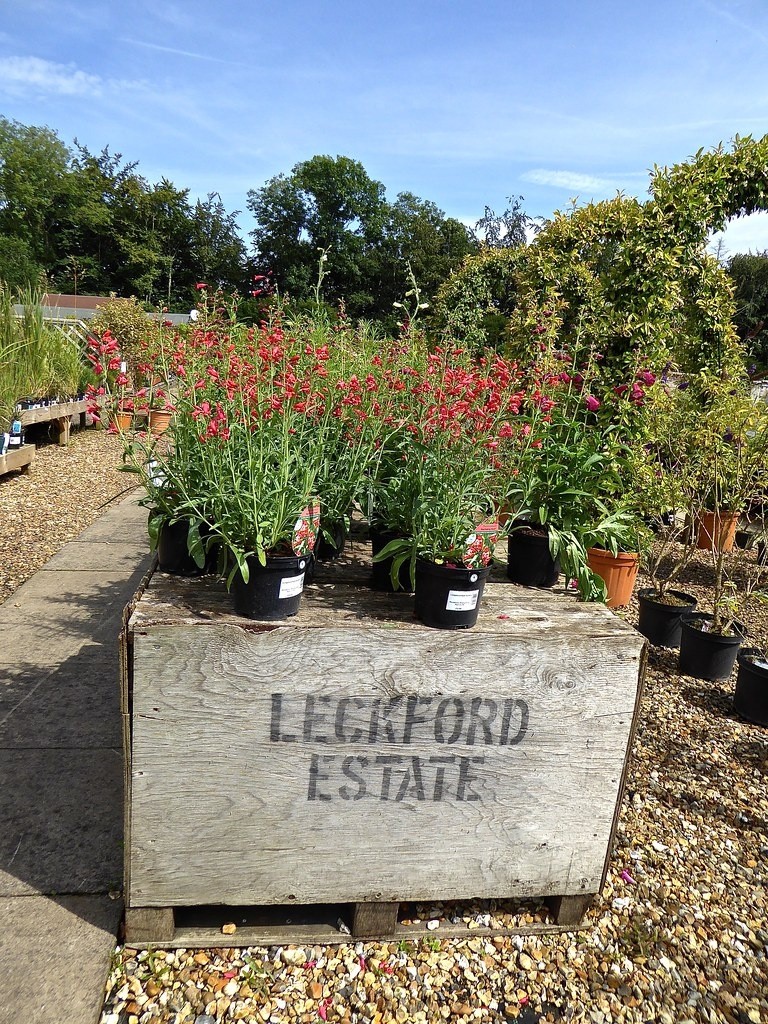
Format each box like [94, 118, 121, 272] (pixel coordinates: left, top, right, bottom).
[155, 505, 644, 633]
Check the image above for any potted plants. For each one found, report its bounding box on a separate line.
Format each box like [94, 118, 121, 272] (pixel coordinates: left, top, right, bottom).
[0, 281, 88, 475]
[640, 365, 768, 729]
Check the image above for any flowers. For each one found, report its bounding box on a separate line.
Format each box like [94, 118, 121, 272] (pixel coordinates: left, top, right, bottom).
[85, 272, 658, 568]
